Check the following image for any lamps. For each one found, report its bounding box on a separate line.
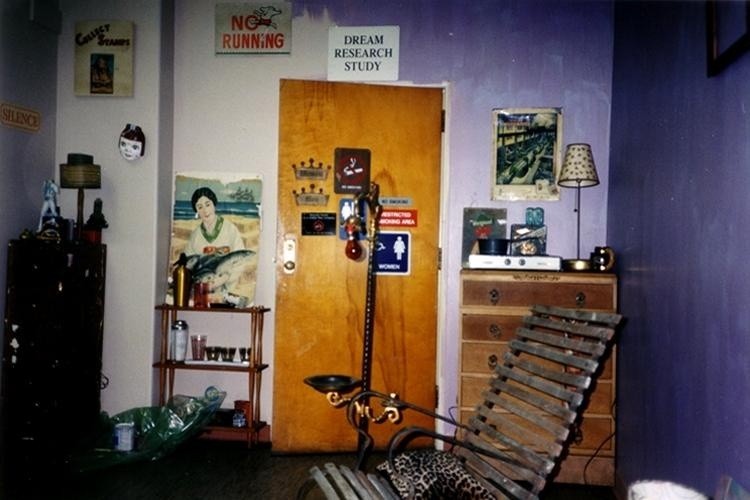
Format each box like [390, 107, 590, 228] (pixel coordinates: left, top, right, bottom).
[558, 143, 600, 272]
[60, 154, 100, 238]
[303, 183, 404, 465]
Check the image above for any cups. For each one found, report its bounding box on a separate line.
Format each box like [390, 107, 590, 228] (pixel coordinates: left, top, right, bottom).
[205, 346, 251, 362]
[190, 334, 207, 361]
[592, 246, 616, 274]
[193, 282, 208, 310]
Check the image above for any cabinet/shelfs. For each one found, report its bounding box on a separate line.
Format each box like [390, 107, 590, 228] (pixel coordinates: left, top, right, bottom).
[2, 239, 107, 442]
[153, 304, 270, 449]
[457, 270, 618, 486]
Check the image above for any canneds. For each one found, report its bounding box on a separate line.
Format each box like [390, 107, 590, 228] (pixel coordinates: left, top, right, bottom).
[113, 422, 134, 452]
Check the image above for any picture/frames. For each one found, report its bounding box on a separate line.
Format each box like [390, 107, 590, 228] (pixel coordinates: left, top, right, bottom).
[706, 0, 750, 78]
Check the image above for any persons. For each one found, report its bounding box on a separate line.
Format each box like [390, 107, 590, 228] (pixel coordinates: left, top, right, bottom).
[185, 187, 246, 294]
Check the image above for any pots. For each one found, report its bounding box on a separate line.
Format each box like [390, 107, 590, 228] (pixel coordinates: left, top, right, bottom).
[473, 236, 537, 253]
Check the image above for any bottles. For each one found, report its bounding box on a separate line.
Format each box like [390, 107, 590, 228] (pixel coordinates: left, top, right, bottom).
[170, 320, 189, 364]
[174, 264, 189, 308]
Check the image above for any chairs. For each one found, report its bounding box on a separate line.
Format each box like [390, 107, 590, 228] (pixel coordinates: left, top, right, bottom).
[298, 305, 624, 500]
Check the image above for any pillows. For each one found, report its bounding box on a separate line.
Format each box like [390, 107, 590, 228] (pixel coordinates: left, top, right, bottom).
[375, 450, 496, 500]
